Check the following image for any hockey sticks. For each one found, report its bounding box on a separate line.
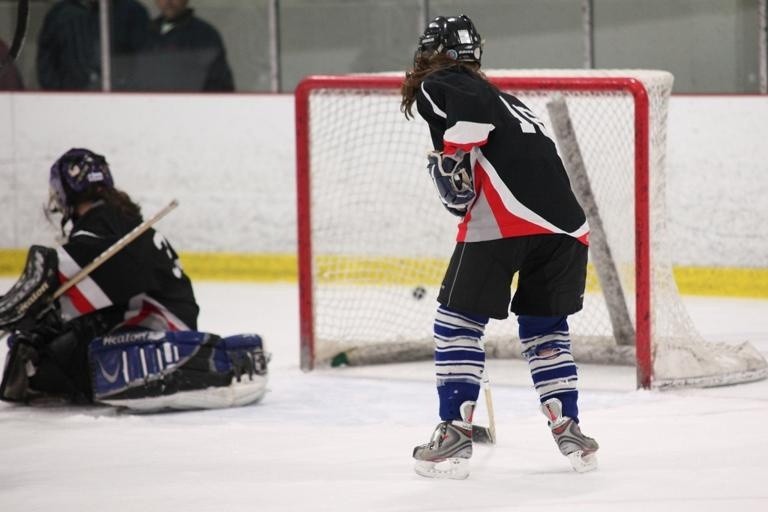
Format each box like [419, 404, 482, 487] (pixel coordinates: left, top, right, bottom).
[471, 352, 497, 447]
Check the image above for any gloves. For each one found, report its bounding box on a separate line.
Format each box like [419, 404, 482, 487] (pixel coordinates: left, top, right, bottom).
[426, 151, 476, 217]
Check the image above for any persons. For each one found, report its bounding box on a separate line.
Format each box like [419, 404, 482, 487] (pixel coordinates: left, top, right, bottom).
[398, 12, 600, 476]
[109, 1, 236, 92]
[8, 147, 267, 410]
[34, 1, 149, 90]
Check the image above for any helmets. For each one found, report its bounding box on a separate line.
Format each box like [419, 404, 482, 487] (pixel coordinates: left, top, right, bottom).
[49, 149, 113, 219]
[413, 14, 483, 76]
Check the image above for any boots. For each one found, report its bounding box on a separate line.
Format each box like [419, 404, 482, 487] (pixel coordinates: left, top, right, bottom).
[413, 420, 472, 461]
[549, 416, 599, 458]
